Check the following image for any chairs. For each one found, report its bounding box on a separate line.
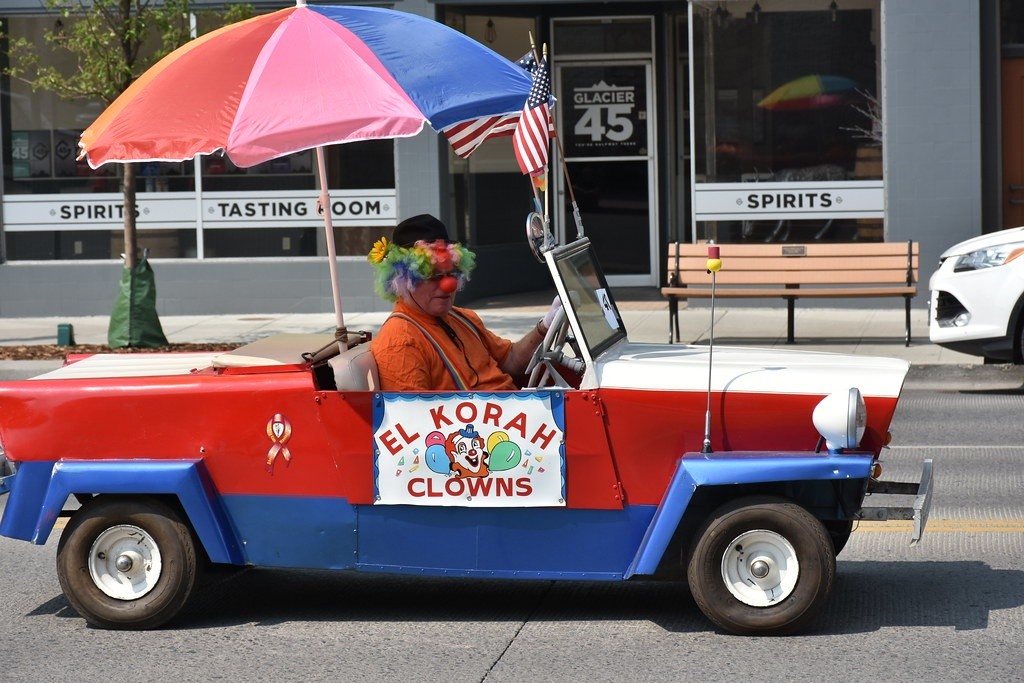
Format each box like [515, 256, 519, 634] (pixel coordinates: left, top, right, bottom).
[326, 341, 380, 391]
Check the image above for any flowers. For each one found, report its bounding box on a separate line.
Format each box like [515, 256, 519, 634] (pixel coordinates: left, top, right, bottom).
[368, 236, 476, 297]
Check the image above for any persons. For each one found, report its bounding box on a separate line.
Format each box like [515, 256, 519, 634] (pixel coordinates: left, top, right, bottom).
[367, 214, 580, 392]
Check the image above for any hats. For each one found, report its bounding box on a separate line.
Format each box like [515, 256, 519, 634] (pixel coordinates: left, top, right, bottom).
[392, 214, 456, 248]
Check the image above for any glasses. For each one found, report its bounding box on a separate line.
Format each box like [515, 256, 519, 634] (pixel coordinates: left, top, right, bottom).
[426, 270, 463, 282]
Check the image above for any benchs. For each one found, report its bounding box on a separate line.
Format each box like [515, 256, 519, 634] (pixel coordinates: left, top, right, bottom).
[661, 242, 919, 347]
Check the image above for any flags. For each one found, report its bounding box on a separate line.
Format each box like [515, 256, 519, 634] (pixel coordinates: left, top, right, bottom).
[512, 54, 555, 175]
[442, 49, 554, 159]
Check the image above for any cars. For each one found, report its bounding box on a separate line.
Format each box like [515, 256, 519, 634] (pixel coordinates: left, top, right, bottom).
[925, 224, 1024, 362]
[0, 235, 936, 639]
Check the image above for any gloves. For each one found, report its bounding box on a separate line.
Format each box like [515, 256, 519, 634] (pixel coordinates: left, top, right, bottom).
[542, 291, 580, 331]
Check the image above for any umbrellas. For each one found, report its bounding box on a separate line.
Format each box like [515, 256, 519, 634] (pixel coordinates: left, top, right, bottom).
[75, 0, 557, 354]
[757, 73, 863, 113]
